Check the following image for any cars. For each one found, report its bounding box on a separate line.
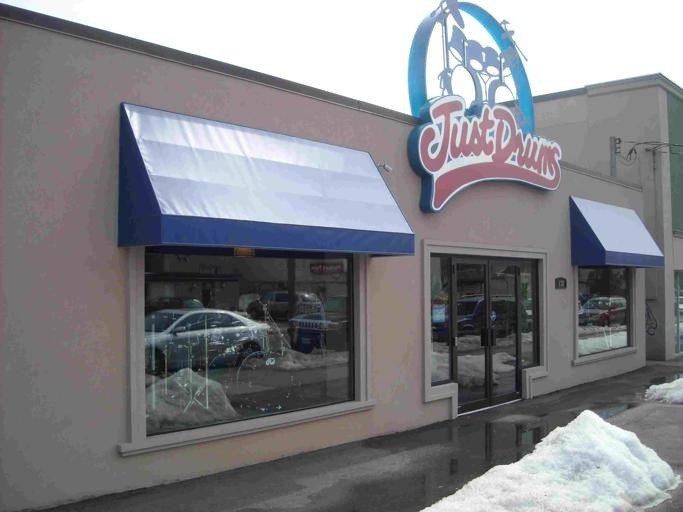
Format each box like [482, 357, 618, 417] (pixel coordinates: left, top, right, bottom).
[246, 290, 322, 320]
[679, 296, 683, 313]
[146, 308, 272, 374]
[146, 296, 205, 313]
[578, 297, 625, 326]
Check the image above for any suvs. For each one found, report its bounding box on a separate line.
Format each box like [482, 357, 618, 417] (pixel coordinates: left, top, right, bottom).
[432, 295, 529, 340]
[287, 293, 351, 352]
